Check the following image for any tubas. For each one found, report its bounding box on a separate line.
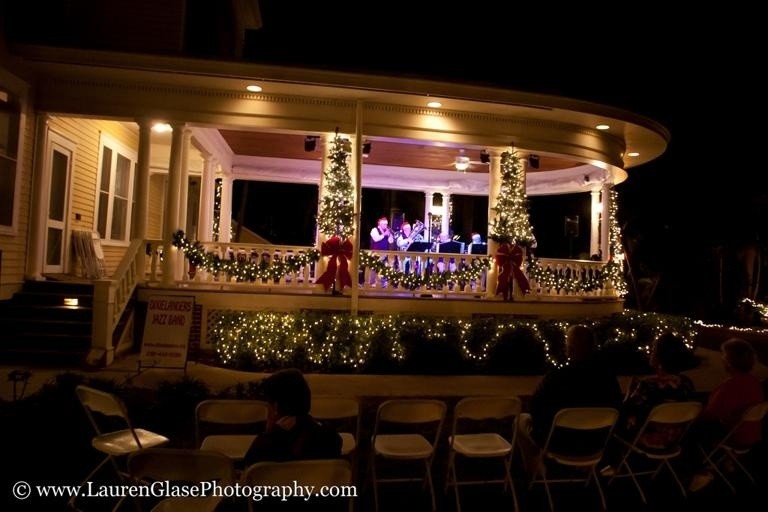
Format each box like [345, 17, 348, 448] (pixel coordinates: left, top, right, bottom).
[400, 219, 426, 251]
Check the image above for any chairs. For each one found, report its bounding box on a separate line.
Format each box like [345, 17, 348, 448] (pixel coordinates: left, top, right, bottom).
[70, 380, 768, 511]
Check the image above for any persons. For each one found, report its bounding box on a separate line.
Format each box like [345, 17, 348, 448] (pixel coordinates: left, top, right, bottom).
[468, 231, 486, 291]
[594, 334, 699, 492]
[395, 220, 424, 289]
[430, 231, 457, 272]
[364, 216, 395, 289]
[510, 323, 623, 484]
[242, 368, 344, 511]
[678, 337, 767, 497]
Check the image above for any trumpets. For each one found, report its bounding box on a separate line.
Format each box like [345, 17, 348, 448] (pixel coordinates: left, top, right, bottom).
[385, 226, 401, 239]
